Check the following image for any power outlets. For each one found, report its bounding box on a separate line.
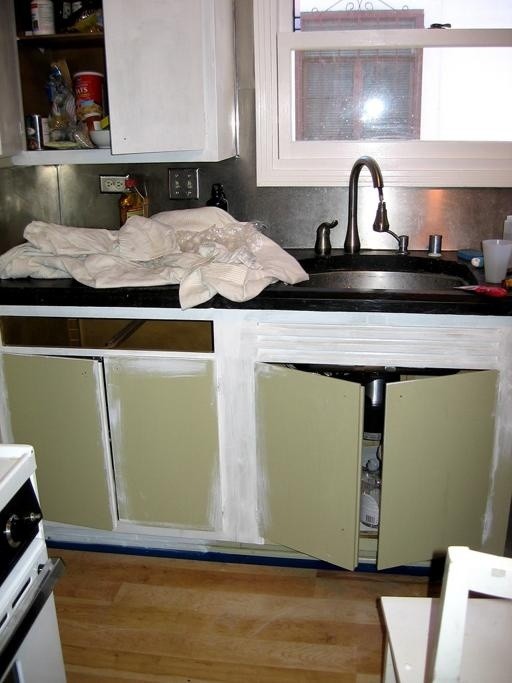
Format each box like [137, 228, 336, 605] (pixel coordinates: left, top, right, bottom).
[168, 166, 201, 200]
[99, 171, 130, 195]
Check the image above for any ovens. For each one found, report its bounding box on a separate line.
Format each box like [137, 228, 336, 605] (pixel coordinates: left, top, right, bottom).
[2, 544, 69, 683]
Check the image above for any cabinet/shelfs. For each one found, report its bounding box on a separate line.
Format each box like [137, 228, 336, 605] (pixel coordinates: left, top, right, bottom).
[0, 0, 242, 171]
[1, 305, 236, 561]
[236, 309, 510, 572]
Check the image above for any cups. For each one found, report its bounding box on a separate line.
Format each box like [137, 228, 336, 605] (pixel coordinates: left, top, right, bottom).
[481, 240, 511, 283]
[73, 71, 103, 131]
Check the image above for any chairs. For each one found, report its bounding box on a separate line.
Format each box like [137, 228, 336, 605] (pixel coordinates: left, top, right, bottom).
[375, 542, 512, 683]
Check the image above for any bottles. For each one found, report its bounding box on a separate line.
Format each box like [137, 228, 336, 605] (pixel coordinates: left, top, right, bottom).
[136, 176, 148, 217]
[119, 179, 144, 226]
[503, 214, 512, 269]
[30, 0, 87, 36]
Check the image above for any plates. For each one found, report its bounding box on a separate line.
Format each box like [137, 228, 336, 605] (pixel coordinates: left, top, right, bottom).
[45, 143, 78, 149]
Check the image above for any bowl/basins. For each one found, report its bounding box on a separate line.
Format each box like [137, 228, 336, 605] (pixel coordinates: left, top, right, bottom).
[90, 130, 111, 149]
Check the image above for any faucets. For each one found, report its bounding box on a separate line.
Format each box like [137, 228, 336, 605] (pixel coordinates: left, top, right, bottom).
[342, 155, 384, 252]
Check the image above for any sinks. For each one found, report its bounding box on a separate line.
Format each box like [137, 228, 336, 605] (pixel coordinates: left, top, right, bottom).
[292, 269, 471, 295]
